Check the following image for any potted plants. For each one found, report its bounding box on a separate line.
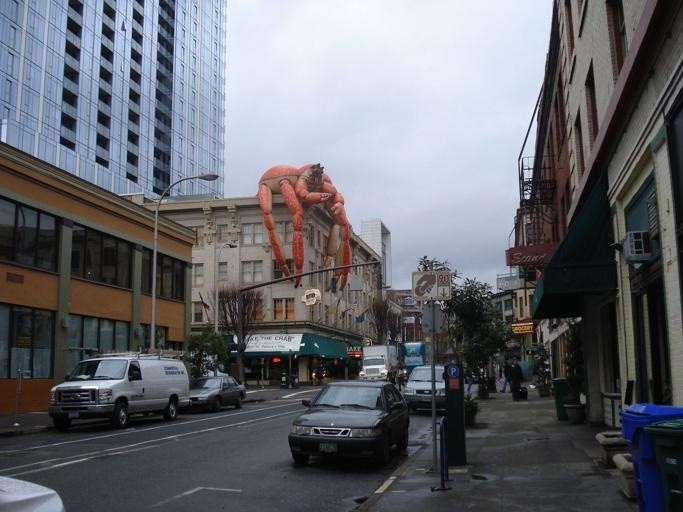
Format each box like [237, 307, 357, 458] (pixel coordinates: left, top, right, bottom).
[563, 376, 584, 425]
[532, 346, 552, 396]
[464, 376, 497, 425]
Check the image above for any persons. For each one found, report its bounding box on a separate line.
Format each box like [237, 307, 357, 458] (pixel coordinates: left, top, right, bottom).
[499, 361, 512, 393]
[506, 356, 523, 401]
[388, 366, 396, 385]
[397, 366, 405, 391]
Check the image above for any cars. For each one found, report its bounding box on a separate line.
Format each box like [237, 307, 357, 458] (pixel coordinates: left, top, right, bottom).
[465, 364, 489, 384]
[181, 373, 247, 414]
[0, 473, 64, 511]
[286, 380, 410, 469]
[405, 364, 450, 413]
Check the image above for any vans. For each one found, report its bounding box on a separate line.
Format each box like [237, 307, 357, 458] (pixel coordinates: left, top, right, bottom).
[47, 350, 191, 429]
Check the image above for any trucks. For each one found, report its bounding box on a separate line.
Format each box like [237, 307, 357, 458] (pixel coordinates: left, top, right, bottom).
[359, 346, 398, 384]
[400, 342, 425, 372]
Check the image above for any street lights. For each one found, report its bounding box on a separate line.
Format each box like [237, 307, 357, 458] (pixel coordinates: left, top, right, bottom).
[147, 171, 222, 356]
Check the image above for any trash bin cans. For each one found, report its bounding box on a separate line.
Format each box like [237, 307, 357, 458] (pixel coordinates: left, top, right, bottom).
[552, 377, 578, 422]
[280, 373, 299, 389]
[621, 403, 683, 512]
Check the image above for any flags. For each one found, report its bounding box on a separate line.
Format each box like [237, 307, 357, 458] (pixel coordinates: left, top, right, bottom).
[323, 297, 339, 314]
[342, 300, 356, 315]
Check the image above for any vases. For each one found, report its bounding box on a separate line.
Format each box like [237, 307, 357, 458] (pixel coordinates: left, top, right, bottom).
[611, 453, 637, 500]
[595, 429, 628, 467]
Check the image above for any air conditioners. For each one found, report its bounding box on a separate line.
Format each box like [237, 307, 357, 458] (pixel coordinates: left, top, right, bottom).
[622, 230, 651, 264]
